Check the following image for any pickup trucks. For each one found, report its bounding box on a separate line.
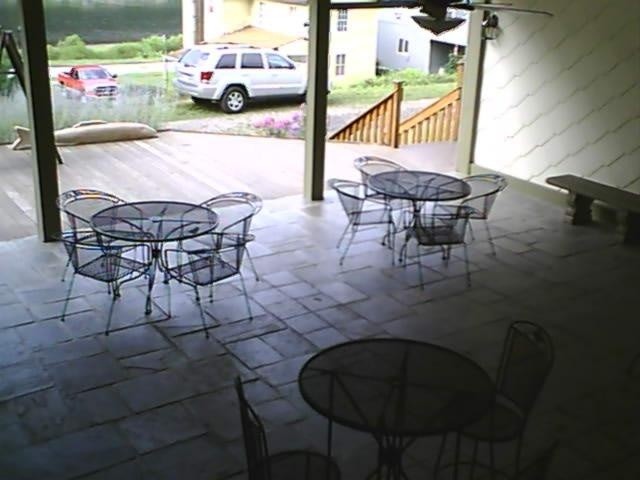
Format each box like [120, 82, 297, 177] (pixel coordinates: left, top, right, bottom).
[58, 64, 123, 108]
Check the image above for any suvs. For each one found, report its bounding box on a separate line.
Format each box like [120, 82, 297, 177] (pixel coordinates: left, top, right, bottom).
[173, 46, 332, 114]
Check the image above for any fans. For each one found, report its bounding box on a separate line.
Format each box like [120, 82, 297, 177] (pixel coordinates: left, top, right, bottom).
[330, 0, 555, 18]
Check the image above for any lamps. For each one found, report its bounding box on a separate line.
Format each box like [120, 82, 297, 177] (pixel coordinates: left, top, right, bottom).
[411, 8, 466, 35]
[483, 15, 498, 39]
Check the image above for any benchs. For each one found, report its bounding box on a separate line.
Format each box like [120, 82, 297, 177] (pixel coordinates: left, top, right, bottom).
[546, 174, 640, 245]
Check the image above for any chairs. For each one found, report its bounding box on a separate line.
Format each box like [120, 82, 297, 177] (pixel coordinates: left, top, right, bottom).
[178, 191, 263, 281]
[433, 320, 555, 480]
[433, 173, 507, 255]
[234, 377, 340, 480]
[354, 155, 426, 225]
[403, 204, 475, 291]
[164, 231, 256, 339]
[51, 231, 148, 336]
[328, 179, 396, 266]
[57, 190, 147, 294]
